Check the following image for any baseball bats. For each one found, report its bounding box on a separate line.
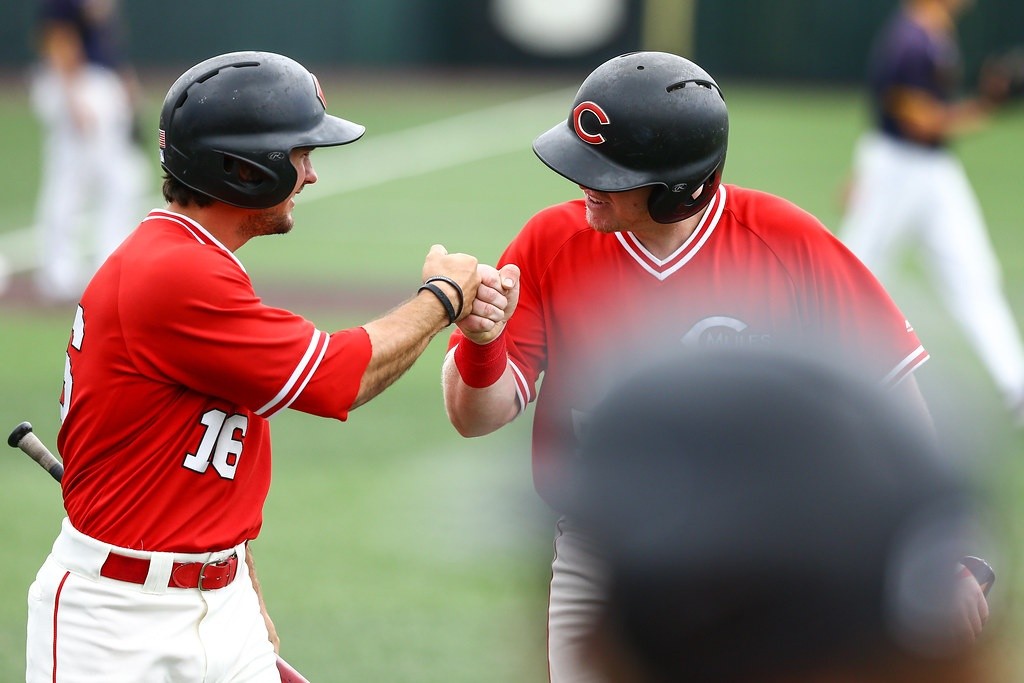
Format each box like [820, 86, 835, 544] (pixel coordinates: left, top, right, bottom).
[8, 421, 311, 683]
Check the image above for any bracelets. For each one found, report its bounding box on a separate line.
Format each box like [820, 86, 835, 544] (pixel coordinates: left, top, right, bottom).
[419, 285, 455, 327]
[425, 277, 464, 320]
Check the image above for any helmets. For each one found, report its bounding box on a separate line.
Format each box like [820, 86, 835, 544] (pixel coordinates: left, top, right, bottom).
[561, 352, 987, 683]
[157, 50, 367, 209]
[531, 52, 729, 223]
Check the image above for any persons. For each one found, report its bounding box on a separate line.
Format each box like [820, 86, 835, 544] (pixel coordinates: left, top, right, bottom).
[0, 2, 151, 310]
[24, 51, 481, 682]
[443, 51, 992, 682]
[840, 0, 1022, 408]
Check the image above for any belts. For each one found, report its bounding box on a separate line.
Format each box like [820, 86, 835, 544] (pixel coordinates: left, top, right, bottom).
[100, 540, 249, 591]
